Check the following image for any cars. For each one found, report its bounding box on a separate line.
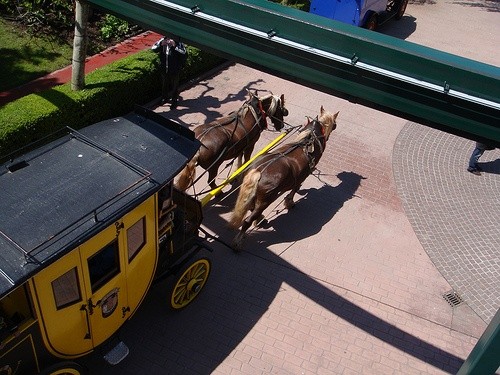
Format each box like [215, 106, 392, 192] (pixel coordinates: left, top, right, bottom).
[309, 0, 409, 34]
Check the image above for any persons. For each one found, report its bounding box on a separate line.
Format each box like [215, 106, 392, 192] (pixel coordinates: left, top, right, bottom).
[152, 36, 187, 110]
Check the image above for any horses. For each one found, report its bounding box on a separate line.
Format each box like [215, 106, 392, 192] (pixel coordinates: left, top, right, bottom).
[174, 93, 288, 198]
[226, 105, 341, 252]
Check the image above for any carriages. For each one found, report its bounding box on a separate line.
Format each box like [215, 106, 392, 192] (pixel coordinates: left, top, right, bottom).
[1, 91, 339, 375]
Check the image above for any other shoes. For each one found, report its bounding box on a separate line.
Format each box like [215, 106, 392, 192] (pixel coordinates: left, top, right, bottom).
[468, 168, 481, 176]
[476, 165, 482, 171]
